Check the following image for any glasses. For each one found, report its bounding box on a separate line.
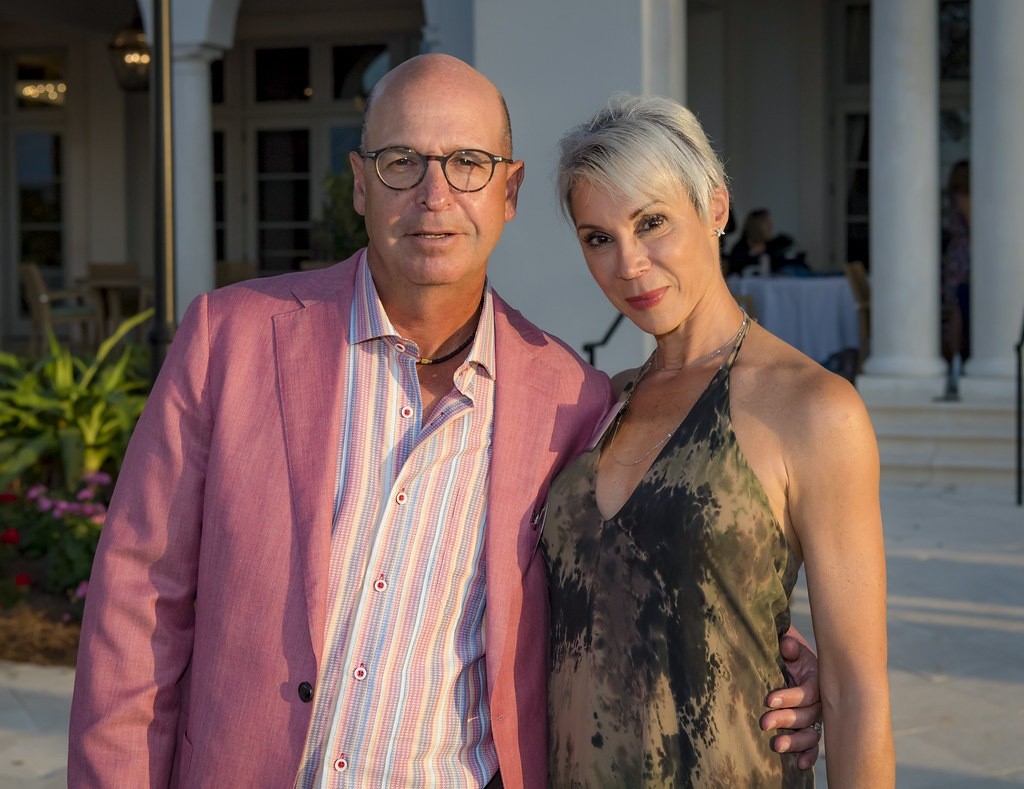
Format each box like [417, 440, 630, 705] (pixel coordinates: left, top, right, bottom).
[358, 145, 514, 193]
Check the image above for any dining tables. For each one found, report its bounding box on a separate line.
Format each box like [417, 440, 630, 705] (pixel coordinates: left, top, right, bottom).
[726, 274, 863, 382]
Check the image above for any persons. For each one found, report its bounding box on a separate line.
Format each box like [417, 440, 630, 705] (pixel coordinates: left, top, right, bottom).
[63, 55, 823, 788]
[939, 157, 972, 376]
[527, 94, 898, 789]
[730, 206, 786, 275]
[842, 137, 870, 374]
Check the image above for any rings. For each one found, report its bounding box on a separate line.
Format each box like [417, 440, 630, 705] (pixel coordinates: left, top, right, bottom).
[810, 721, 823, 742]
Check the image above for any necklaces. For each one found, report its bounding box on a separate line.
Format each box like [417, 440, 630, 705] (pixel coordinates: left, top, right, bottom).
[655, 318, 745, 371]
[415, 329, 476, 365]
[608, 320, 746, 464]
